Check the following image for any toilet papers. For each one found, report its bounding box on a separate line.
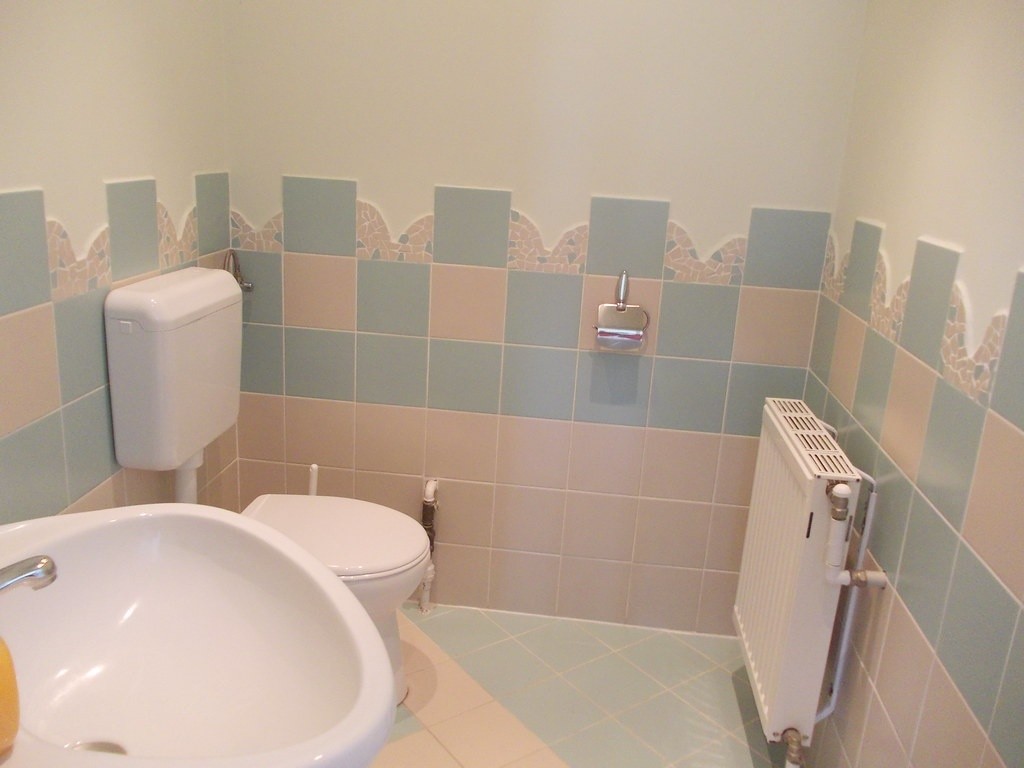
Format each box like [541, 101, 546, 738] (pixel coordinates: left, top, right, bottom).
[601, 337, 645, 352]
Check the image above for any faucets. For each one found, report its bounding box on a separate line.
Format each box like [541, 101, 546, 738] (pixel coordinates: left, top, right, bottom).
[1, 555, 58, 591]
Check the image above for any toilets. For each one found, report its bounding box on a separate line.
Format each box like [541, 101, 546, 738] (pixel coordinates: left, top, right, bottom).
[104, 268, 431, 708]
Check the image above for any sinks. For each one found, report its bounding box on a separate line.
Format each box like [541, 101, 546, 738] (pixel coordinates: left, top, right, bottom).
[1, 503, 396, 768]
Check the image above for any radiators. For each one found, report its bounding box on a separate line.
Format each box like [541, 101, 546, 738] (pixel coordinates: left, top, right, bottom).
[733, 397, 887, 768]
